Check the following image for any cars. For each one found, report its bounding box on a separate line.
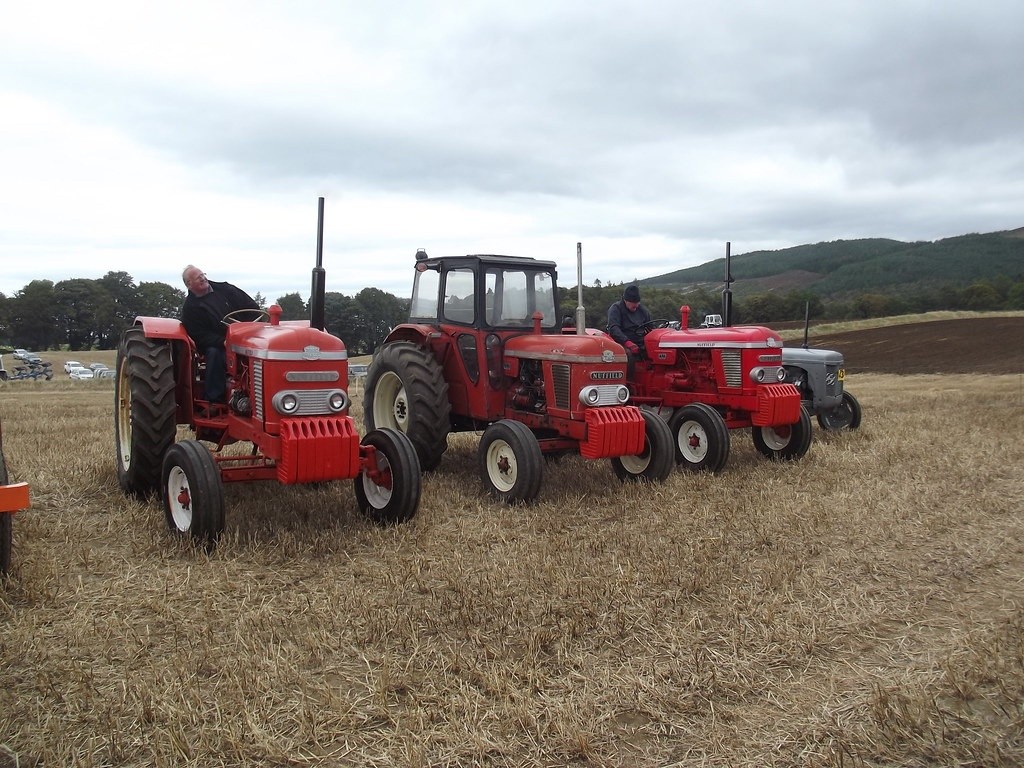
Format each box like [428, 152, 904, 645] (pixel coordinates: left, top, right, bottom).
[23, 355, 42, 364]
[63, 360, 115, 380]
[13, 349, 28, 359]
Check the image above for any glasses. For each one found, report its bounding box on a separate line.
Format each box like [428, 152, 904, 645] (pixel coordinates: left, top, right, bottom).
[190, 272, 207, 283]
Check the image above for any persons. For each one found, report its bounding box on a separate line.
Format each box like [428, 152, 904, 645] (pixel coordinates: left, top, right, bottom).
[181, 265, 262, 419]
[607, 286, 653, 395]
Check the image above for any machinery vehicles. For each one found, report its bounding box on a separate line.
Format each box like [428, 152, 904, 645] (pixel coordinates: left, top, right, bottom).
[564, 241, 813, 474]
[360, 241, 676, 504]
[114, 196, 422, 556]
[661, 300, 862, 433]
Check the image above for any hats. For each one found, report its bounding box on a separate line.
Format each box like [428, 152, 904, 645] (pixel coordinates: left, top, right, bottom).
[623, 285, 641, 303]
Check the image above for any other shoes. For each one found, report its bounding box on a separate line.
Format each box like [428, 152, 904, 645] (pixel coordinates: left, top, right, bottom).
[200, 402, 220, 417]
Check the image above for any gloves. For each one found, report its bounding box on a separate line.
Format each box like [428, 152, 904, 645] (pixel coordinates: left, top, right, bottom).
[623, 340, 640, 354]
